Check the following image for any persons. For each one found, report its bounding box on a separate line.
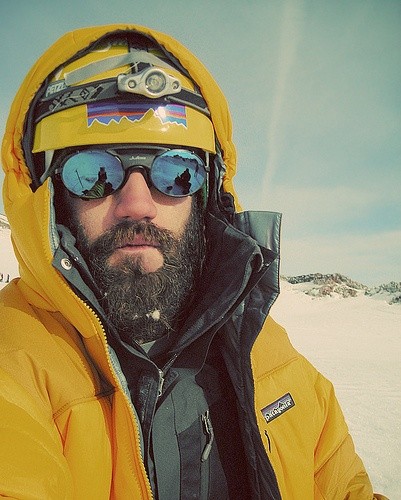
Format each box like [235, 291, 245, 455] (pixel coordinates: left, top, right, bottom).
[82, 171, 107, 198]
[167, 170, 192, 196]
[0, 23, 389, 500]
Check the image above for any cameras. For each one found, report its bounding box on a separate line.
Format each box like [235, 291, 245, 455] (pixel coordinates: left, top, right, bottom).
[100, 167, 105, 175]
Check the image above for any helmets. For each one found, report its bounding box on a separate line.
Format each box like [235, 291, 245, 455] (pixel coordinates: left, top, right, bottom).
[30, 32, 217, 157]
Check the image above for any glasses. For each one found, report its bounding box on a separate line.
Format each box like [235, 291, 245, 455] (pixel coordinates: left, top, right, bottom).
[53, 147, 210, 199]
